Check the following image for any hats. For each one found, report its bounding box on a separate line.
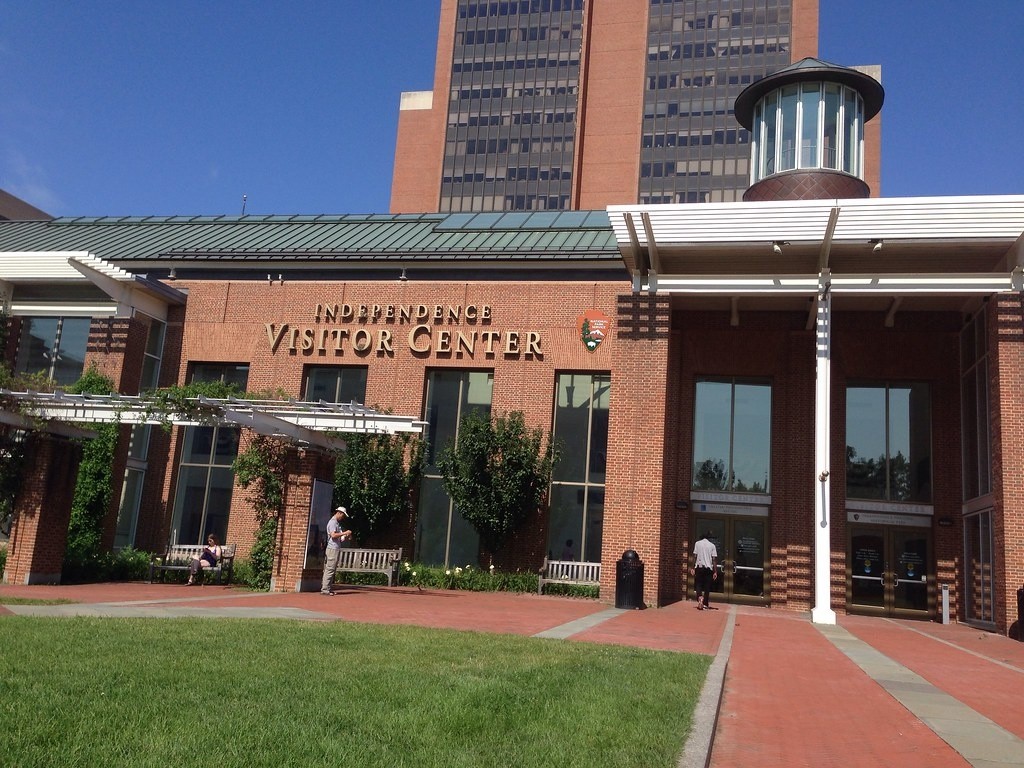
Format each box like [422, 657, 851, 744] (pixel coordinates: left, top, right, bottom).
[336, 507, 349, 518]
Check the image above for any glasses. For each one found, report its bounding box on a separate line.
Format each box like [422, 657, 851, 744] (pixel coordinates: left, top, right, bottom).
[208, 539, 213, 542]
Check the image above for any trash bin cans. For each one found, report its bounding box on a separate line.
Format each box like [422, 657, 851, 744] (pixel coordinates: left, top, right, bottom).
[1017, 583, 1024, 642]
[615, 549, 644, 609]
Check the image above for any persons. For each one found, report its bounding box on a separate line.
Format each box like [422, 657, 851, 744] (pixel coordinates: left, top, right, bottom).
[563, 539, 573, 560]
[690, 528, 717, 610]
[321, 506, 352, 594]
[185, 534, 221, 586]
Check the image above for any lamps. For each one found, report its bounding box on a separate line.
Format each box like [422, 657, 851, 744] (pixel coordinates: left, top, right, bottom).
[772, 240, 791, 255]
[868, 239, 884, 252]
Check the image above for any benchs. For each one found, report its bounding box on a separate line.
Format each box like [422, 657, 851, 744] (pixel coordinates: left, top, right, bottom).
[537, 555, 601, 596]
[335, 547, 403, 587]
[150, 543, 237, 585]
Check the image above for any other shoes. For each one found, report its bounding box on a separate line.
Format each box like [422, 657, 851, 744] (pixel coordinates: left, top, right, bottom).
[698, 596, 705, 609]
[704, 604, 709, 608]
[185, 581, 194, 585]
